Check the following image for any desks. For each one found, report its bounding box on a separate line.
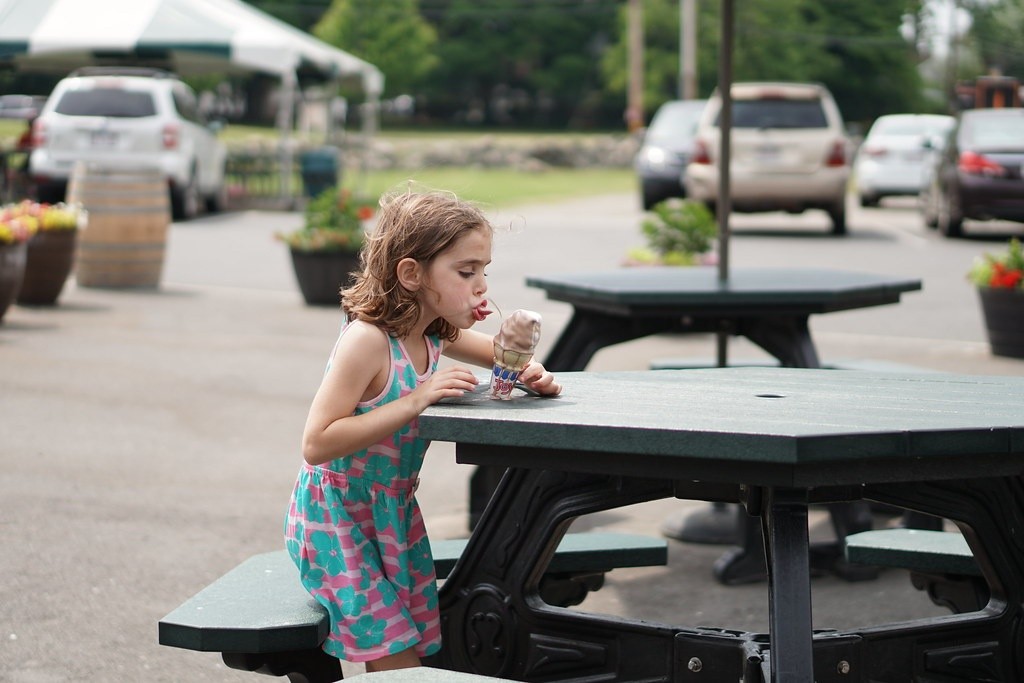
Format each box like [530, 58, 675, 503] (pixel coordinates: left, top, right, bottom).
[417, 368, 1024, 683]
[523, 264, 925, 368]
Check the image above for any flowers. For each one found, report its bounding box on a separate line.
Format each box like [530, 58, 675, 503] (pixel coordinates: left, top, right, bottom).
[0, 199, 90, 244]
[275, 183, 375, 252]
[964, 236, 1024, 289]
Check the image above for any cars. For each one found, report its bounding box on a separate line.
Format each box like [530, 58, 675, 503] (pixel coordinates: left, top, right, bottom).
[630, 96, 707, 211]
[1, 93, 44, 122]
[852, 113, 957, 207]
[679, 83, 856, 237]
[919, 106, 1024, 237]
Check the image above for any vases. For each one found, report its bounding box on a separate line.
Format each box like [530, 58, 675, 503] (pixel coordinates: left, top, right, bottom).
[14, 228, 75, 307]
[975, 286, 1024, 361]
[0, 239, 28, 318]
[288, 247, 361, 309]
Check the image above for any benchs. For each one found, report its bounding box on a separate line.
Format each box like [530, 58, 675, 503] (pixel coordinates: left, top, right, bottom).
[157, 531, 670, 682]
[844, 525, 991, 615]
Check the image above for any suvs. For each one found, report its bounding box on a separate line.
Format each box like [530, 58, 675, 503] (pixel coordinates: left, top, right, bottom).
[26, 65, 231, 221]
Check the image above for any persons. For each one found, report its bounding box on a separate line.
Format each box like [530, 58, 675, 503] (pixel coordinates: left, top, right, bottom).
[283, 179, 562, 672]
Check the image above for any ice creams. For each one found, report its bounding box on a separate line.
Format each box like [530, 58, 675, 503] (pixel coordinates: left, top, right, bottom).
[493, 309, 542, 374]
